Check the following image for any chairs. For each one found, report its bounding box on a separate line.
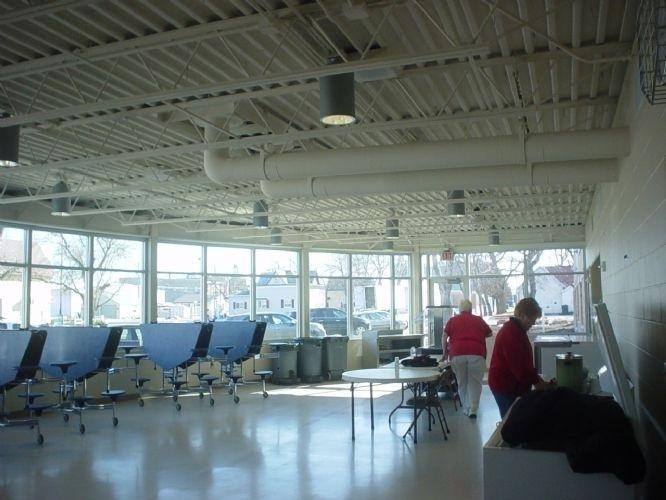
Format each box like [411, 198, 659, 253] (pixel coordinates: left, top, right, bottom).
[402, 369, 451, 441]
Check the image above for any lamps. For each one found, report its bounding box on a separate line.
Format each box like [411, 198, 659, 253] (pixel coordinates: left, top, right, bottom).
[253, 199, 268, 230]
[50, 181, 71, 216]
[319, 56, 356, 126]
[381, 241, 394, 252]
[270, 227, 281, 245]
[447, 189, 465, 218]
[488, 225, 499, 246]
[385, 207, 400, 239]
[0, 113, 19, 168]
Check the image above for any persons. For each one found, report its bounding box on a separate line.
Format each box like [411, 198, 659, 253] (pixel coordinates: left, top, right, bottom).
[441, 298, 492, 419]
[488, 296, 547, 420]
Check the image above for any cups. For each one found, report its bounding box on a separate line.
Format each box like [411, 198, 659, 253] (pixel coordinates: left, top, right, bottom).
[394, 357, 399, 366]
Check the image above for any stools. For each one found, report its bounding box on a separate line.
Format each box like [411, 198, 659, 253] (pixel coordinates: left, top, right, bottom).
[95, 357, 121, 393]
[24, 403, 58, 445]
[68, 396, 98, 434]
[192, 372, 210, 399]
[216, 346, 234, 359]
[200, 378, 219, 406]
[245, 345, 264, 372]
[165, 374, 184, 392]
[125, 355, 148, 388]
[226, 375, 243, 403]
[118, 346, 136, 369]
[101, 391, 126, 426]
[17, 394, 45, 429]
[254, 372, 273, 398]
[131, 378, 151, 407]
[168, 381, 189, 411]
[13, 366, 43, 410]
[191, 348, 208, 376]
[222, 370, 238, 395]
[51, 362, 77, 401]
[53, 390, 77, 422]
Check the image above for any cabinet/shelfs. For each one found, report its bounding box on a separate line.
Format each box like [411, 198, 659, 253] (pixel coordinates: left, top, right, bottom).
[362, 329, 424, 369]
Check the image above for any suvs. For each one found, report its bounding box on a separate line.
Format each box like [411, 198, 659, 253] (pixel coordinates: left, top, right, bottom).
[290, 308, 371, 336]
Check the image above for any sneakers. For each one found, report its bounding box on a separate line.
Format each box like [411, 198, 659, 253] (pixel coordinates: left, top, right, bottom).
[469, 410, 477, 418]
[462, 406, 468, 414]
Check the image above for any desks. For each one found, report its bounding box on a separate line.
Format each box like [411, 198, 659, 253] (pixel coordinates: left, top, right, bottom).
[0, 330, 47, 428]
[208, 322, 267, 394]
[139, 324, 213, 402]
[341, 368, 438, 444]
[29, 326, 123, 422]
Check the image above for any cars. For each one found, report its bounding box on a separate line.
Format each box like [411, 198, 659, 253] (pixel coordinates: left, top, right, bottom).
[227, 312, 326, 341]
[356, 310, 408, 331]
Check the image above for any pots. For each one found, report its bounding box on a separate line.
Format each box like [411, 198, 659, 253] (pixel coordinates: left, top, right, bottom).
[552, 352, 584, 389]
